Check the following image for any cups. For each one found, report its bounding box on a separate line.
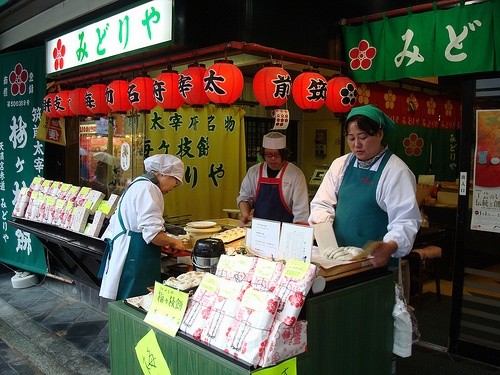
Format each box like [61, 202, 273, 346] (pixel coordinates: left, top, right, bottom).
[425, 195, 436, 206]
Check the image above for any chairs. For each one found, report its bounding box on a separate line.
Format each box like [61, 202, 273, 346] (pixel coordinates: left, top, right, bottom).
[409, 223, 446, 310]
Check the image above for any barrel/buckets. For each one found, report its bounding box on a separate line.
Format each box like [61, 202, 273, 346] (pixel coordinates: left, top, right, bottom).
[189, 238, 226, 275]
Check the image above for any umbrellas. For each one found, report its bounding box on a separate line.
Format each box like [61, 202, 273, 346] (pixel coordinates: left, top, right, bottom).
[92, 152, 115, 165]
[79, 148, 87, 155]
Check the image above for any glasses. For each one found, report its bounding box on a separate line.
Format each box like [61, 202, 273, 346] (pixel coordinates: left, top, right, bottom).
[265, 154, 281, 159]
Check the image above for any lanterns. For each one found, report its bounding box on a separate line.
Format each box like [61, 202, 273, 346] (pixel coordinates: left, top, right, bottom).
[42, 59, 359, 117]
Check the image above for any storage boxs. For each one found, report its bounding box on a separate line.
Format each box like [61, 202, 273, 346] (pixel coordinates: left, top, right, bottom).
[175, 250, 319, 370]
[11, 175, 119, 240]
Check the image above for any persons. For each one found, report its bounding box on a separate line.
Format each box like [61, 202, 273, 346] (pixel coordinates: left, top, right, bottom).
[80, 156, 107, 183]
[236, 131, 309, 225]
[98, 153, 184, 301]
[309, 103, 423, 266]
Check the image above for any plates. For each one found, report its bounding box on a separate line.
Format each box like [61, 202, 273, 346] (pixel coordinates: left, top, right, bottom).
[216, 227, 247, 243]
[186, 221, 217, 228]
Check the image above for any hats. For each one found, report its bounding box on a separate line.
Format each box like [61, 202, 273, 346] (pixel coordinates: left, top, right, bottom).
[144, 154, 184, 185]
[263, 132, 286, 149]
